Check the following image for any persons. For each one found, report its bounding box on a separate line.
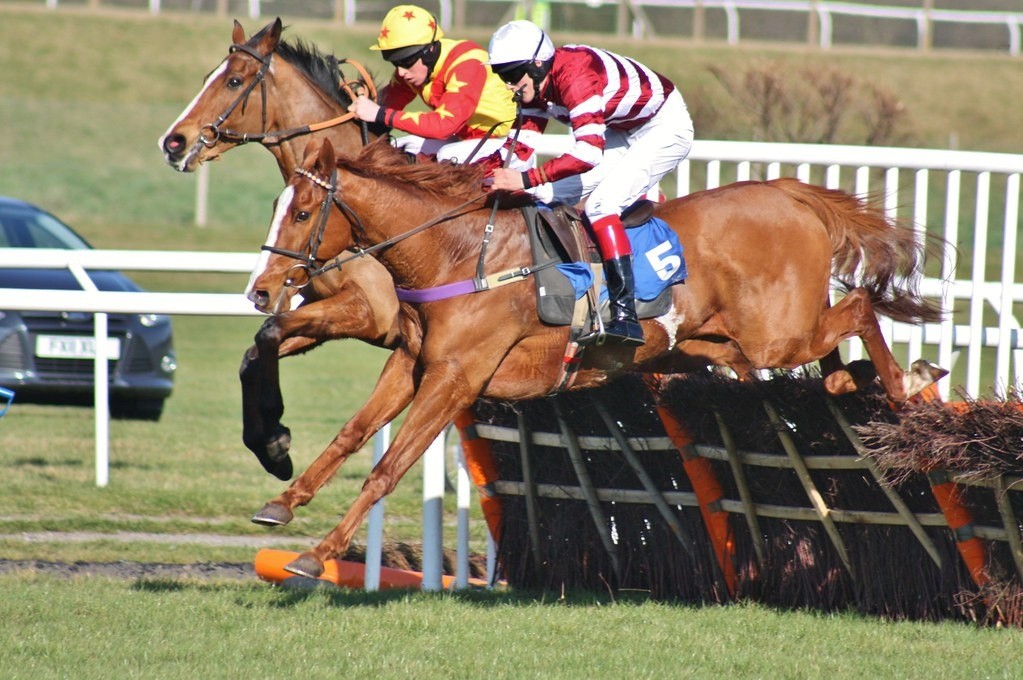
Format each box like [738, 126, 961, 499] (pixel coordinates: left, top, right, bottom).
[345, 5, 518, 167]
[473, 20, 696, 346]
[498, 0, 551, 33]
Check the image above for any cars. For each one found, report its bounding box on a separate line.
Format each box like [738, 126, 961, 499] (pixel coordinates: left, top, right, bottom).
[0, 198, 175, 422]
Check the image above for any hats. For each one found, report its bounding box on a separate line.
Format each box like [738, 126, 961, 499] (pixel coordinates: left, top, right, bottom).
[482, 19, 556, 66]
[368, 5, 444, 51]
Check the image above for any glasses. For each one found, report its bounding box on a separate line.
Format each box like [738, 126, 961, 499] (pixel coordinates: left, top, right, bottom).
[498, 59, 536, 85]
[390, 49, 428, 68]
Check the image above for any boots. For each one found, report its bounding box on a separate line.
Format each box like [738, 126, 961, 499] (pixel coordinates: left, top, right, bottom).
[576, 257, 646, 349]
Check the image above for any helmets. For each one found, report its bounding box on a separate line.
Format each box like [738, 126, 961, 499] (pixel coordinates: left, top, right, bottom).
[380, 44, 424, 61]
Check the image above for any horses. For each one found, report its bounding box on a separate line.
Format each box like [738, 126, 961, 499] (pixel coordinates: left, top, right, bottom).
[159, 17, 856, 481]
[248, 136, 948, 605]
